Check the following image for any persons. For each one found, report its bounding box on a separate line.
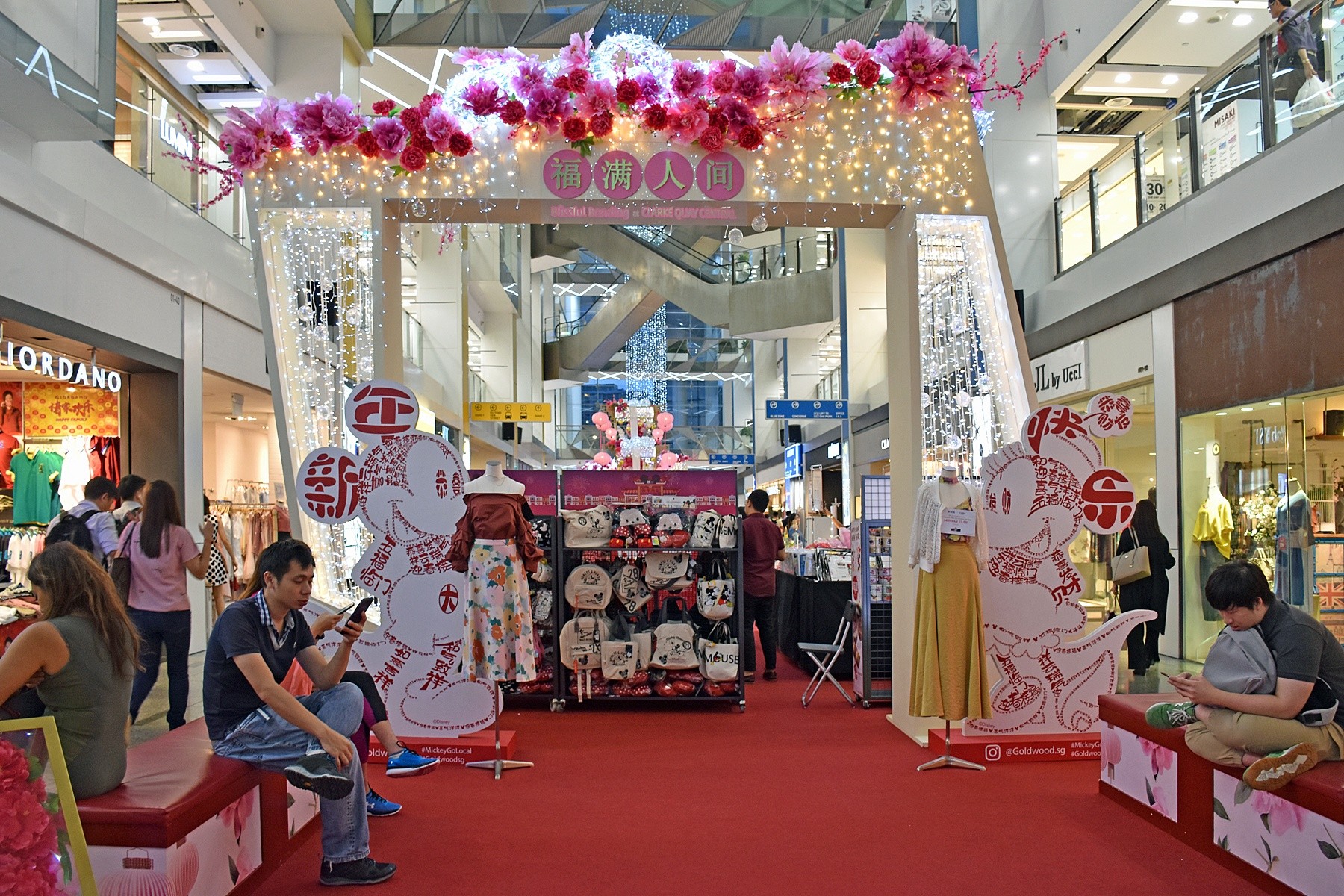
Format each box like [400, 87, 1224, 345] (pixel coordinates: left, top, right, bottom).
[1110, 500, 1176, 675]
[0, 391, 23, 434]
[1267, 0, 1318, 130]
[908, 465, 993, 722]
[741, 489, 786, 684]
[823, 509, 851, 530]
[0, 541, 144, 799]
[203, 540, 396, 886]
[446, 461, 543, 684]
[764, 511, 806, 548]
[1275, 478, 1319, 605]
[47, 476, 238, 744]
[1145, 561, 1344, 792]
[1192, 485, 1234, 622]
[238, 550, 440, 816]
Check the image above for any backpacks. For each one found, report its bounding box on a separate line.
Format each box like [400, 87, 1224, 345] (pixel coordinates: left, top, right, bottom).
[45, 509, 104, 553]
[1202, 625, 1277, 694]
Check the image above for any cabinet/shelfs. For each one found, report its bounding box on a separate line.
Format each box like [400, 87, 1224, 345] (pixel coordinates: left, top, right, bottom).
[558, 511, 746, 712]
[850, 520, 896, 707]
[498, 516, 556, 707]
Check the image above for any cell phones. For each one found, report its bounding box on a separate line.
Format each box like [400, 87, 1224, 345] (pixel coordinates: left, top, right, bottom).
[338, 602, 354, 614]
[341, 598, 373, 635]
[1160, 672, 1169, 678]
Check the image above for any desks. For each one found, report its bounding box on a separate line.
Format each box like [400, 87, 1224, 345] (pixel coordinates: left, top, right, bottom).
[773, 570, 852, 682]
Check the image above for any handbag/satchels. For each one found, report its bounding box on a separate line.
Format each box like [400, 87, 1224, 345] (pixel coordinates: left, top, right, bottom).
[560, 504, 612, 547]
[715, 513, 738, 549]
[108, 520, 140, 606]
[1102, 593, 1126, 651]
[1111, 526, 1151, 585]
[688, 508, 721, 549]
[528, 517, 552, 620]
[559, 550, 739, 681]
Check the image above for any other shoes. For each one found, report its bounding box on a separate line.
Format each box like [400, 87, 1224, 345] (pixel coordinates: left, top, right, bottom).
[745, 675, 755, 682]
[1147, 659, 1159, 669]
[762, 670, 777, 681]
[1133, 668, 1146, 676]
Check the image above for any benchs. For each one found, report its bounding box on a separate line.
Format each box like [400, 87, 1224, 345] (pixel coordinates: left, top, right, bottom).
[1098, 692, 1344, 896]
[53, 717, 321, 896]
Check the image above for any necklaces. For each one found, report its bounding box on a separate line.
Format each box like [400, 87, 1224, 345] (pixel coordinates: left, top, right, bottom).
[939, 476, 958, 484]
[486, 474, 506, 479]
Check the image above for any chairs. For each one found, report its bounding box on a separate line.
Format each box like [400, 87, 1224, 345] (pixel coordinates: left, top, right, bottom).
[797, 599, 857, 708]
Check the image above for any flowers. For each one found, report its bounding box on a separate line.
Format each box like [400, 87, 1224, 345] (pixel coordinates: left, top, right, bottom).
[162, 20, 1067, 209]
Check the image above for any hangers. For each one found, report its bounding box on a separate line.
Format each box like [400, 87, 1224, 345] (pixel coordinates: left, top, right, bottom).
[210, 479, 280, 521]
[0, 520, 47, 537]
[10, 439, 63, 461]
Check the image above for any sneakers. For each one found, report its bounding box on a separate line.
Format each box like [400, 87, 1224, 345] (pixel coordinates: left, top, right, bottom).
[1145, 702, 1198, 729]
[284, 751, 354, 800]
[319, 855, 397, 885]
[365, 783, 402, 816]
[1243, 743, 1318, 791]
[386, 740, 440, 777]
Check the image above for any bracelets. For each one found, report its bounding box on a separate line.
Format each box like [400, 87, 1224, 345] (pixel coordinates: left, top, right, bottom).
[316, 634, 325, 639]
[1302, 59, 1309, 63]
[830, 515, 834, 519]
